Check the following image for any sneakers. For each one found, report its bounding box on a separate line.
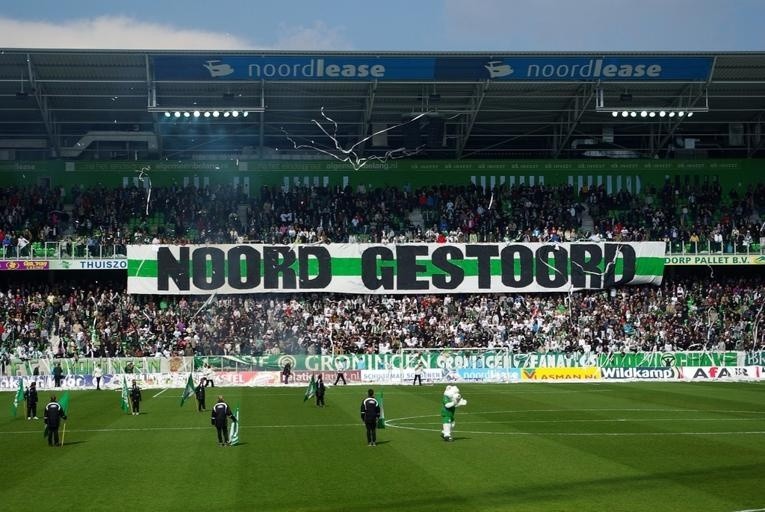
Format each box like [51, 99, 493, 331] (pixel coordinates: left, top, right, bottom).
[216, 441, 232, 447]
[367, 442, 377, 448]
[131, 411, 141, 417]
[440, 432, 453, 442]
[27, 416, 40, 421]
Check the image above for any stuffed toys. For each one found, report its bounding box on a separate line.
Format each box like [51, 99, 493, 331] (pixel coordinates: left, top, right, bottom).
[440, 385, 469, 441]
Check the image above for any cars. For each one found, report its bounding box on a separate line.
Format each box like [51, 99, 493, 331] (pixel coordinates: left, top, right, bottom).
[483, 60, 514, 79]
[201, 59, 235, 78]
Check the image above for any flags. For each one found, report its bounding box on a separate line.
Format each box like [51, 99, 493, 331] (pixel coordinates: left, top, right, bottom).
[178, 372, 194, 407]
[11, 376, 22, 418]
[120, 374, 130, 414]
[374, 384, 386, 429]
[303, 373, 316, 401]
[41, 388, 68, 435]
[229, 397, 240, 446]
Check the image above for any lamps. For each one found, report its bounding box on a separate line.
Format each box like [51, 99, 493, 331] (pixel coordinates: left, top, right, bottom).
[147, 87, 266, 119]
[595, 87, 709, 120]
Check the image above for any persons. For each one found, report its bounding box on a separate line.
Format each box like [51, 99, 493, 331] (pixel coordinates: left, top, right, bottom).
[205, 366, 214, 387]
[333, 358, 347, 385]
[194, 378, 207, 411]
[51, 363, 64, 387]
[92, 364, 103, 391]
[315, 376, 326, 406]
[412, 359, 424, 385]
[32, 364, 41, 375]
[129, 385, 142, 416]
[0, 179, 764, 374]
[282, 361, 295, 383]
[360, 389, 381, 446]
[43, 394, 67, 446]
[23, 382, 39, 420]
[210, 395, 236, 447]
